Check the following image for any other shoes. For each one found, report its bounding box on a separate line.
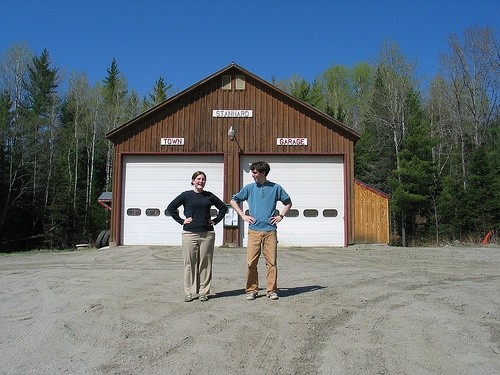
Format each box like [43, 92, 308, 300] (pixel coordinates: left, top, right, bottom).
[246, 293, 256, 299]
[266, 291, 279, 300]
[199, 294, 208, 301]
[184, 294, 194, 302]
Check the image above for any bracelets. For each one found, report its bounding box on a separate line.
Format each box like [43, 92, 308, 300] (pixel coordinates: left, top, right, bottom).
[279, 214, 284, 220]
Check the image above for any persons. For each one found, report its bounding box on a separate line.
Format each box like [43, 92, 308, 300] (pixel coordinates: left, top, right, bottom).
[166, 170, 229, 302]
[229, 161, 292, 300]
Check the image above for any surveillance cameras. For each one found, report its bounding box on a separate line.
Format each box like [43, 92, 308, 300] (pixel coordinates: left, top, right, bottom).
[227, 125, 235, 141]
